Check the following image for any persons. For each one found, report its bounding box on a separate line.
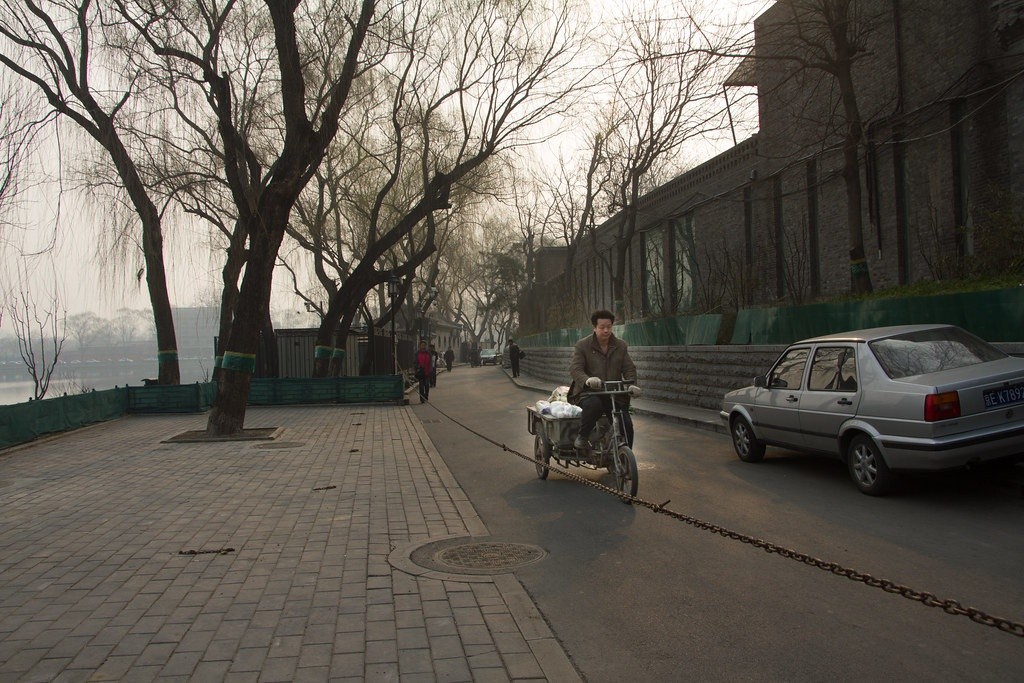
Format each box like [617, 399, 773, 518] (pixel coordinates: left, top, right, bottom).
[444, 346, 454, 371]
[429, 344, 438, 387]
[508, 339, 520, 377]
[471, 346, 482, 366]
[414, 341, 432, 402]
[568, 310, 641, 447]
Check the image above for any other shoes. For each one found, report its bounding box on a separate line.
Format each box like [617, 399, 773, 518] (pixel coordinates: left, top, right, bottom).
[420, 400, 424, 404]
[574, 433, 587, 449]
[622, 465, 630, 479]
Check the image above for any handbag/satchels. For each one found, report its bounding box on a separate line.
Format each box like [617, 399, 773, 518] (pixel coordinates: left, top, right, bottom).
[519, 351, 525, 360]
[415, 367, 423, 379]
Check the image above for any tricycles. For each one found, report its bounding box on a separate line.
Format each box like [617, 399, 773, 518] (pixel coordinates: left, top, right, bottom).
[524, 379, 640, 506]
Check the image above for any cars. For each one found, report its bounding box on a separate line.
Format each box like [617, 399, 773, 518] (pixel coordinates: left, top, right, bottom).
[479, 349, 503, 366]
[718, 321, 1024, 498]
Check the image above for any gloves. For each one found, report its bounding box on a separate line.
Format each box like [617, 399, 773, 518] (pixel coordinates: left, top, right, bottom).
[628, 385, 642, 398]
[585, 377, 603, 388]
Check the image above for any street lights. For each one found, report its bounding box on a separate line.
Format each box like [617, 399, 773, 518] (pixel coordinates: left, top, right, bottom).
[416, 302, 424, 352]
[386, 275, 400, 375]
